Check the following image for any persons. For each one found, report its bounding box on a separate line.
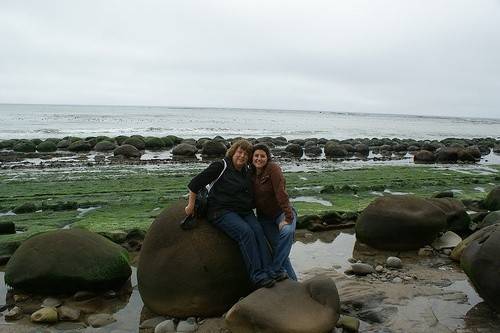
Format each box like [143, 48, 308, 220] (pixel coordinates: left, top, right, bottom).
[251, 143, 297, 280]
[184, 139, 288, 288]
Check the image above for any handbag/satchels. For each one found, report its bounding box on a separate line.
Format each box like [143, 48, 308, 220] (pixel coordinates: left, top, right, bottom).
[180, 187, 209, 230]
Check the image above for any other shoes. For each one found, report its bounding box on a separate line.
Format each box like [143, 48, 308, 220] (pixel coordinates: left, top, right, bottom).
[258, 279, 276, 287]
[275, 273, 285, 282]
